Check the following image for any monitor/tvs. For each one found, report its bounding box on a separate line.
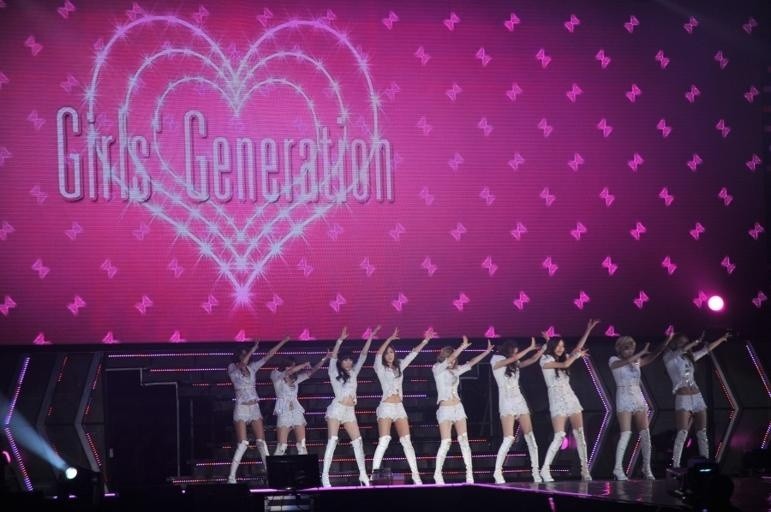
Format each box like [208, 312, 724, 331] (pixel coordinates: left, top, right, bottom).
[266, 454, 320, 496]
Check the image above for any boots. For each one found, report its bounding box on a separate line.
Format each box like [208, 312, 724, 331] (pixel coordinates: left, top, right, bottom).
[457, 432, 475, 484]
[672, 429, 688, 469]
[695, 428, 709, 459]
[370, 435, 392, 481]
[350, 436, 370, 486]
[493, 435, 515, 484]
[399, 434, 422, 485]
[572, 427, 593, 481]
[256, 439, 270, 472]
[612, 431, 633, 481]
[639, 429, 656, 480]
[541, 431, 566, 482]
[524, 431, 542, 483]
[321, 436, 339, 487]
[228, 441, 250, 484]
[433, 439, 452, 485]
[296, 442, 307, 455]
[273, 443, 288, 456]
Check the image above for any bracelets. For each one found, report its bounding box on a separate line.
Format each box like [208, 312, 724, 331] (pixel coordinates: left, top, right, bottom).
[422, 339, 429, 344]
[322, 357, 327, 362]
[337, 338, 343, 345]
[572, 351, 582, 359]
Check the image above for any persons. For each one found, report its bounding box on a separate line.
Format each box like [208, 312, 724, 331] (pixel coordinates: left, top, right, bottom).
[431, 333, 495, 484]
[226, 335, 293, 484]
[270, 348, 334, 456]
[608, 323, 675, 481]
[539, 318, 602, 483]
[490, 330, 549, 484]
[664, 325, 734, 477]
[320, 325, 383, 487]
[369, 324, 437, 485]
